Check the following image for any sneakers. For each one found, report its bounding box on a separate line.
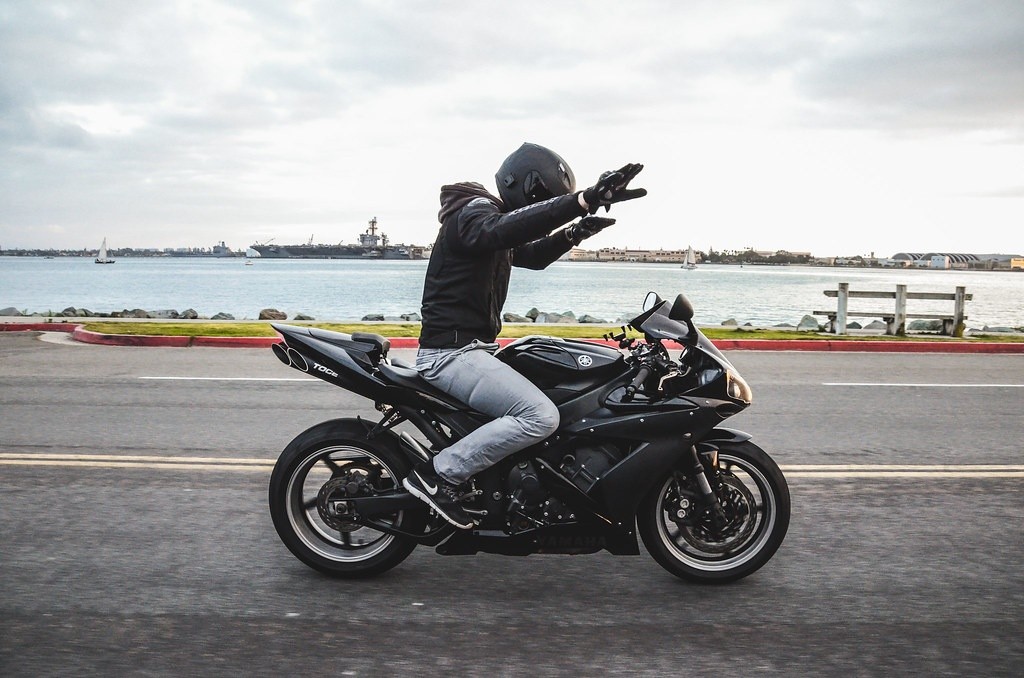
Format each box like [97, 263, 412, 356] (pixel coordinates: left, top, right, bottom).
[402, 463, 473, 530]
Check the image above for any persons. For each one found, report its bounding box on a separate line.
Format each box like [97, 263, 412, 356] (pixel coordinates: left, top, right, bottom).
[402, 141, 647, 529]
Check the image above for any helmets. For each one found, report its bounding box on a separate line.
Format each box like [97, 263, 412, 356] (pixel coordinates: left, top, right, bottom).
[496, 142, 576, 213]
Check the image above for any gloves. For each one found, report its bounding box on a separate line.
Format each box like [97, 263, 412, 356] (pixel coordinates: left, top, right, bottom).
[565, 216, 616, 246]
[583, 163, 647, 212]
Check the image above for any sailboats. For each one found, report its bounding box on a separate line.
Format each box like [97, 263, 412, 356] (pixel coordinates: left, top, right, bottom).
[680, 245, 698, 269]
[94, 237, 117, 264]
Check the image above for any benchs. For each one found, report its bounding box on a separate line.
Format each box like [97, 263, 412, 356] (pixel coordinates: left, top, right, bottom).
[814, 282, 973, 337]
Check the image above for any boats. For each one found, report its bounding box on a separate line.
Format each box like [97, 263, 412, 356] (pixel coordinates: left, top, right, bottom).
[168, 241, 238, 257]
[249, 217, 424, 260]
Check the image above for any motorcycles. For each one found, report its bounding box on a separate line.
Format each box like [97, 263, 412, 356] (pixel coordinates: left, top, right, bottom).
[266, 293, 793, 587]
